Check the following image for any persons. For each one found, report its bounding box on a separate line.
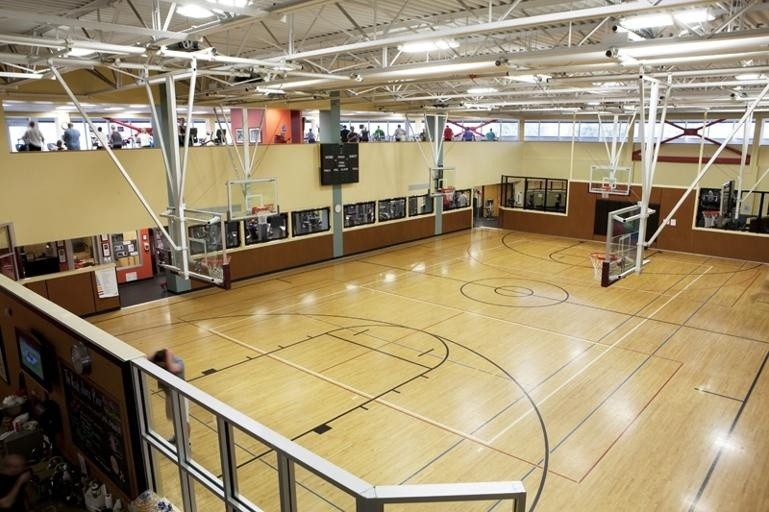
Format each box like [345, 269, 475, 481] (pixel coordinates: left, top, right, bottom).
[0, 453, 32, 512]
[14, 116, 155, 152]
[486, 128, 495, 141]
[177, 116, 192, 146]
[306, 121, 407, 141]
[458, 190, 469, 207]
[246, 219, 259, 241]
[444, 125, 453, 140]
[463, 127, 475, 141]
[420, 129, 427, 142]
[147, 348, 192, 449]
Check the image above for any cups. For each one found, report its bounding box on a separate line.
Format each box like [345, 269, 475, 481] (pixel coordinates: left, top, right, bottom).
[12, 419, 21, 432]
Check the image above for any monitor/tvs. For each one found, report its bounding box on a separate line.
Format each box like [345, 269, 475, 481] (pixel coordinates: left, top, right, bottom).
[14, 326, 55, 395]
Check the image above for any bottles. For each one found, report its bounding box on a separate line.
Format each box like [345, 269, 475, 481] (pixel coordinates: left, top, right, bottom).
[2, 417, 12, 431]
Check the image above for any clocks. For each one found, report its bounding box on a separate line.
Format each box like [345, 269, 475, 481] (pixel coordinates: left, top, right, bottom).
[70, 341, 92, 376]
